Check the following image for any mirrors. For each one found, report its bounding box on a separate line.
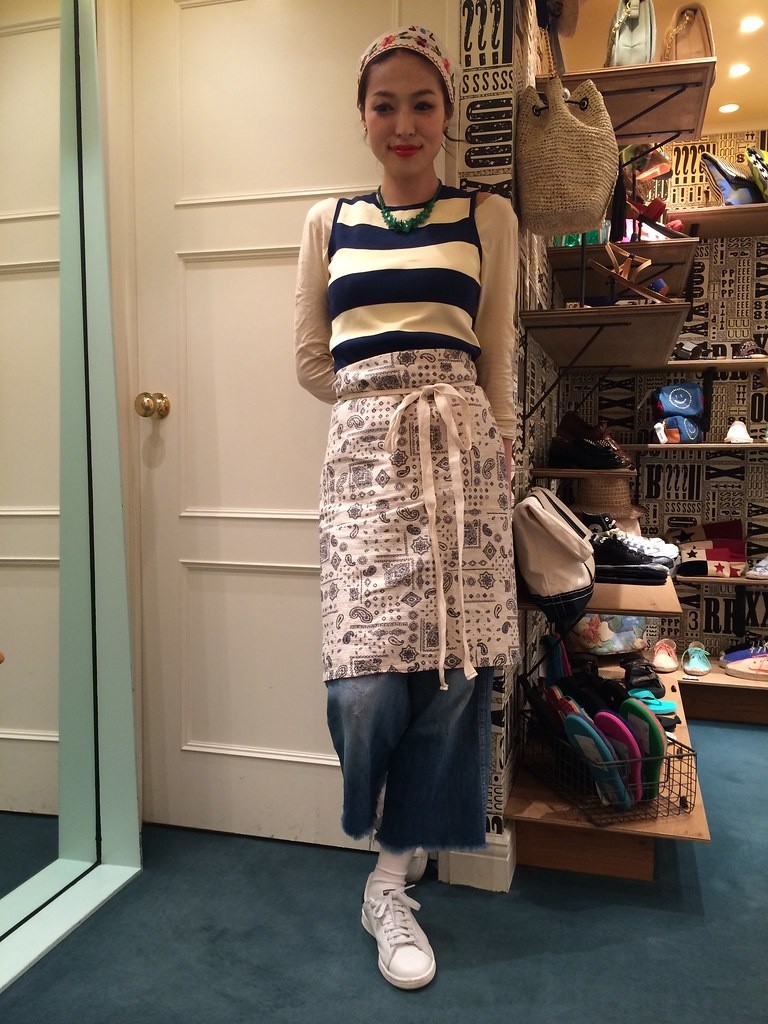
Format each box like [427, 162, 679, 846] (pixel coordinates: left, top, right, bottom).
[0, 0, 145, 996]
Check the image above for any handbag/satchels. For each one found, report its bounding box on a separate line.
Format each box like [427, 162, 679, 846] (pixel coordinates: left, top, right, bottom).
[660, 2, 718, 88]
[650, 416, 703, 444]
[513, 486, 595, 618]
[663, 519, 748, 578]
[605, 1, 657, 67]
[514, 25, 618, 236]
[651, 382, 704, 419]
[584, 242, 675, 304]
[701, 146, 768, 207]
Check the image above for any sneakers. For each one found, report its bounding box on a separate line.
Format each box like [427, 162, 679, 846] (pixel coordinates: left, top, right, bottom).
[579, 509, 680, 585]
[372, 808, 427, 881]
[719, 634, 768, 681]
[361, 873, 436, 989]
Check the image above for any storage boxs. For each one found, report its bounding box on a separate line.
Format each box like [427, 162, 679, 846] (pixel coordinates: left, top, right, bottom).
[515, 709, 698, 828]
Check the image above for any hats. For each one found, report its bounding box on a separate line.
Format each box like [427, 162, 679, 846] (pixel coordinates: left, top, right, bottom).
[358, 25, 455, 110]
[566, 478, 646, 519]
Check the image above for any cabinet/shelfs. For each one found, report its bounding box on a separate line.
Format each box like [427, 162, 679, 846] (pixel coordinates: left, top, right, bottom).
[503, 56, 768, 882]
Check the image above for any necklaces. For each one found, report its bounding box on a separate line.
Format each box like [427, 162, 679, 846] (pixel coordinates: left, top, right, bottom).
[377, 178, 442, 232]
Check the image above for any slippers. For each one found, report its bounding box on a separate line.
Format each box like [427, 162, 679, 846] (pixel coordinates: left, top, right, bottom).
[626, 688, 676, 714]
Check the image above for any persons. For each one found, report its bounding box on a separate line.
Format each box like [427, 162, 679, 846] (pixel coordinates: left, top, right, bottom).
[295, 27, 519, 989]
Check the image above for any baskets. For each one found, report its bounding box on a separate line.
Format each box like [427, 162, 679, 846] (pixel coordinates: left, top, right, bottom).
[517, 709, 697, 826]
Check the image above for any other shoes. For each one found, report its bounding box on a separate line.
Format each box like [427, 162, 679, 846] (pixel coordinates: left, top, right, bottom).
[724, 421, 754, 444]
[547, 412, 633, 469]
[681, 642, 710, 675]
[527, 674, 667, 811]
[746, 555, 768, 579]
[734, 338, 767, 358]
[652, 639, 679, 672]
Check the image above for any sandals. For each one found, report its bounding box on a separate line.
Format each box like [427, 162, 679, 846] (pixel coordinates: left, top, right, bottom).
[571, 652, 600, 680]
[618, 651, 664, 697]
